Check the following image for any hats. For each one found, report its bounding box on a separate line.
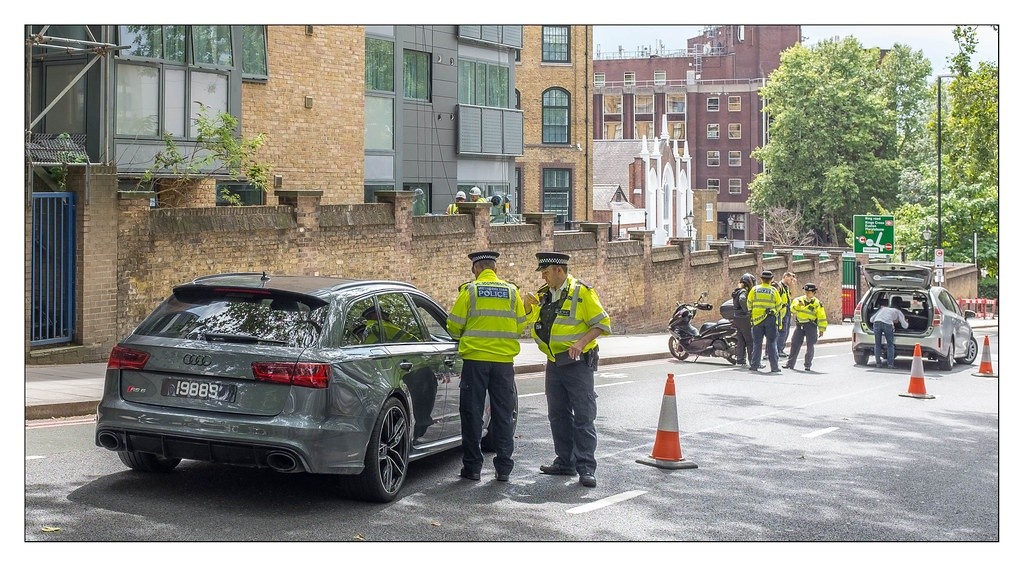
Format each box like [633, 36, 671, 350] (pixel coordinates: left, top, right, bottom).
[534, 252, 571, 271]
[468, 251, 500, 273]
[761, 271, 774, 279]
[802, 283, 817, 291]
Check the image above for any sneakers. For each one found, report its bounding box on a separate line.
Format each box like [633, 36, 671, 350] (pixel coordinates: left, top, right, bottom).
[461, 466, 480, 479]
[495, 471, 509, 480]
[579, 472, 596, 486]
[540, 462, 577, 476]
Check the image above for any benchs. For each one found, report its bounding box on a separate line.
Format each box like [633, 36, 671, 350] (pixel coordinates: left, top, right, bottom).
[867, 299, 928, 331]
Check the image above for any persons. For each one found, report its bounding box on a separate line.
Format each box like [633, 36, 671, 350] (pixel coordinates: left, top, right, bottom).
[523, 253, 614, 487]
[446, 190, 466, 215]
[732, 273, 767, 369]
[870, 304, 909, 369]
[772, 272, 795, 357]
[784, 283, 828, 370]
[469, 186, 487, 203]
[352, 305, 439, 445]
[413, 189, 427, 215]
[746, 270, 782, 372]
[446, 251, 527, 481]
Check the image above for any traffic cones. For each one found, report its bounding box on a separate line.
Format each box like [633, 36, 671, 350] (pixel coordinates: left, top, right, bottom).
[635, 372, 699, 470]
[898, 342, 936, 399]
[970, 334, 998, 378]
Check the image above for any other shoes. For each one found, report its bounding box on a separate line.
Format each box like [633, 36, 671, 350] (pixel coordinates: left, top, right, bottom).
[749, 367, 757, 371]
[736, 363, 747, 366]
[771, 369, 781, 372]
[779, 352, 788, 357]
[876, 364, 883, 367]
[888, 365, 899, 369]
[805, 366, 810, 370]
[782, 365, 793, 369]
[758, 364, 766, 368]
[763, 356, 768, 359]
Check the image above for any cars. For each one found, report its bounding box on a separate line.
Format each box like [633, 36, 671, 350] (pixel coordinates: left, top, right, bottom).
[850, 263, 979, 372]
[94, 271, 519, 504]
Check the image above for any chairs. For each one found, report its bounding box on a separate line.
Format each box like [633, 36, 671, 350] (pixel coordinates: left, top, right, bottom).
[891, 296, 902, 307]
[270, 298, 302, 322]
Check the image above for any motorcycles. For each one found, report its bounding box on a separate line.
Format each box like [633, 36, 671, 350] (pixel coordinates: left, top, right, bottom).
[666, 290, 749, 366]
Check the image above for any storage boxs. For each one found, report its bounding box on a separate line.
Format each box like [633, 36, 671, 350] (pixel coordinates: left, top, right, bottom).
[720, 298, 734, 319]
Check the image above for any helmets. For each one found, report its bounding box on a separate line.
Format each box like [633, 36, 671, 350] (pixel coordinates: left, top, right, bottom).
[456, 191, 466, 199]
[740, 273, 756, 291]
[414, 189, 424, 196]
[469, 187, 481, 196]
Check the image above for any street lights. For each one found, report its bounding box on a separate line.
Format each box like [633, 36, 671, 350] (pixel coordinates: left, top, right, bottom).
[935, 74, 957, 286]
